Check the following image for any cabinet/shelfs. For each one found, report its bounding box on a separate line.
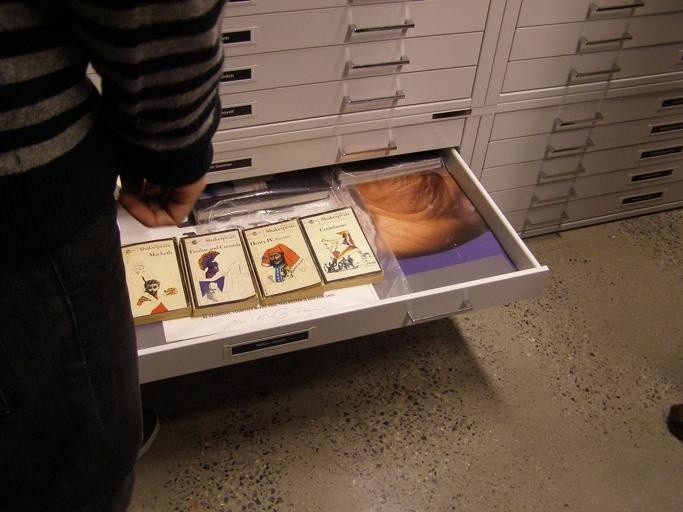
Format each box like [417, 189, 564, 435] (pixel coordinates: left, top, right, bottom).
[469, 0, 683, 256]
[83, 0, 549, 388]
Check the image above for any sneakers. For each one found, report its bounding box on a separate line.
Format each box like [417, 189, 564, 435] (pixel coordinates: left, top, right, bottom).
[666, 401, 683, 442]
[135, 409, 161, 461]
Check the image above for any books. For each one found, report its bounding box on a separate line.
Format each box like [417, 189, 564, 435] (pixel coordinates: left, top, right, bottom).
[179, 228, 259, 318]
[299, 206, 383, 292]
[242, 216, 325, 306]
[333, 148, 443, 188]
[121, 236, 192, 327]
[191, 168, 331, 224]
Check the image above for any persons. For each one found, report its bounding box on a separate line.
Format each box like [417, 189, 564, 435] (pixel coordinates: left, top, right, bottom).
[0, 0, 228, 512]
[136, 277, 178, 317]
[320, 234, 365, 271]
[261, 243, 303, 282]
